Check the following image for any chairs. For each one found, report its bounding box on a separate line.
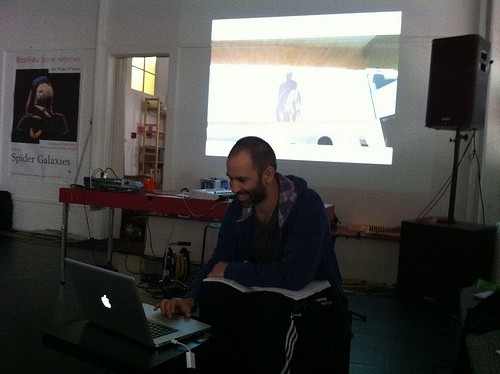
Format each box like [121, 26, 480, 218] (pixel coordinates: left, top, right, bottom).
[338, 261, 367, 321]
[457, 287, 500, 374]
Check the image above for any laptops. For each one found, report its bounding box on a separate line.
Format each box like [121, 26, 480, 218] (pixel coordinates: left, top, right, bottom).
[65, 258, 211, 348]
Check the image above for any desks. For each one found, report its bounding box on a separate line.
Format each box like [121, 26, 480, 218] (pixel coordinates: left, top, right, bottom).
[43, 317, 213, 374]
[59, 190, 334, 286]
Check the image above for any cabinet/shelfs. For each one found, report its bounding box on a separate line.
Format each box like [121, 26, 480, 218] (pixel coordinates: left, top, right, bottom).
[140, 97, 167, 190]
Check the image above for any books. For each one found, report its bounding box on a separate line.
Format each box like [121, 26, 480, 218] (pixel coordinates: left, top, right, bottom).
[203, 276, 332, 300]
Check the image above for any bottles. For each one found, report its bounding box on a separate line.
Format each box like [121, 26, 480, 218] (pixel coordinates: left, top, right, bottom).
[125, 131, 139, 176]
[136, 127, 146, 175]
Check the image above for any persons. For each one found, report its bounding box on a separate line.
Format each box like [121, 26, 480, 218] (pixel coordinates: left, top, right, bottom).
[153, 137, 354, 374]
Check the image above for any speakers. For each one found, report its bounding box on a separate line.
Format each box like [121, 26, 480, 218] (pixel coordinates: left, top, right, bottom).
[425, 33, 491, 131]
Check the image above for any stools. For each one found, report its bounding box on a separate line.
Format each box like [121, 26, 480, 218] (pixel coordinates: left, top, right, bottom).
[201, 223, 221, 268]
